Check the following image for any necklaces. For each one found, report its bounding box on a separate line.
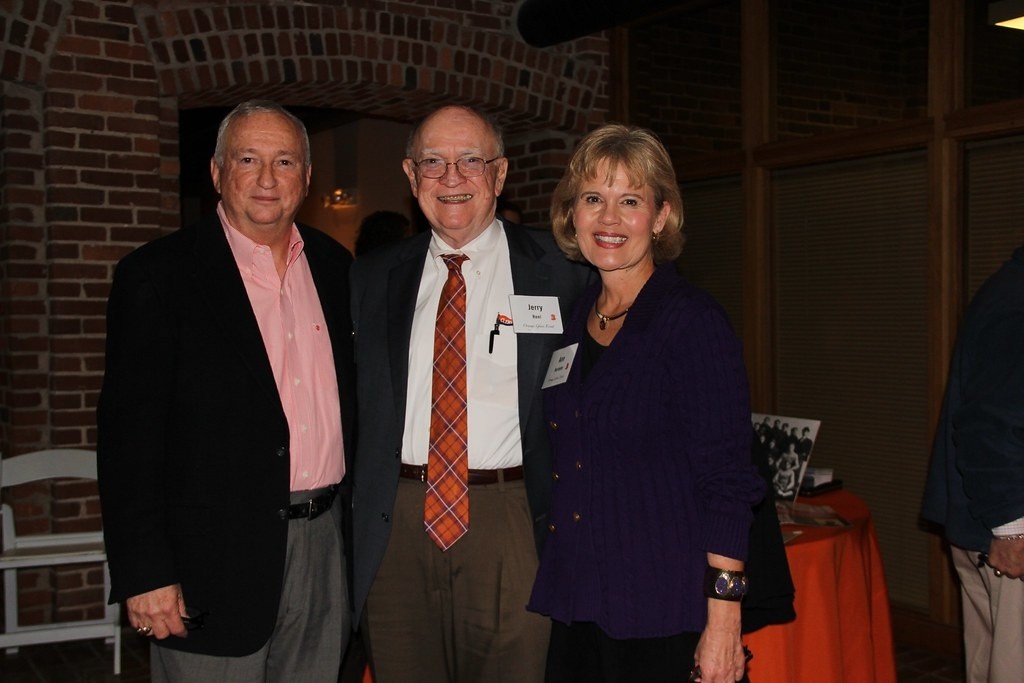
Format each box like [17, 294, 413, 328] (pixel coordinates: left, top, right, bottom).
[595, 293, 629, 330]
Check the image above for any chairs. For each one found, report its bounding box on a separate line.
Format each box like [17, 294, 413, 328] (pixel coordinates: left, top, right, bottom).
[0, 449, 121, 675]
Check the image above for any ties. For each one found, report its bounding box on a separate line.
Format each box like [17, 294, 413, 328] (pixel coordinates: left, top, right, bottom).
[424, 253, 470, 553]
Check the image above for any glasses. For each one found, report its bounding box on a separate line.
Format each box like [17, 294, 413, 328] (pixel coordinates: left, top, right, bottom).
[410, 157, 499, 179]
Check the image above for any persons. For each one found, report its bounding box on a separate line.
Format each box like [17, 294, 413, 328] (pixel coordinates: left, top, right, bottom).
[495, 200, 524, 227]
[919, 239, 1024, 683]
[356, 210, 412, 259]
[754, 417, 813, 499]
[95, 99, 357, 683]
[524, 123, 796, 683]
[349, 104, 601, 683]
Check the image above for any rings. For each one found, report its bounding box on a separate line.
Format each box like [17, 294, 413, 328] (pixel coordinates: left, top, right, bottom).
[137, 625, 152, 636]
[994, 568, 1003, 577]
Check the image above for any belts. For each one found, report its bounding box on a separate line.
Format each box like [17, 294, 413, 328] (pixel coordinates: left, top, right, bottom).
[399, 463, 523, 487]
[287, 484, 341, 522]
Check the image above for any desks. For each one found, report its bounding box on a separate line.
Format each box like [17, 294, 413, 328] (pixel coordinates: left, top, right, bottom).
[742, 488, 897, 683]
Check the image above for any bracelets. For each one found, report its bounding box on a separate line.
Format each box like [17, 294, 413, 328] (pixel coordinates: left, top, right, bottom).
[992, 533, 1024, 542]
[703, 565, 750, 603]
